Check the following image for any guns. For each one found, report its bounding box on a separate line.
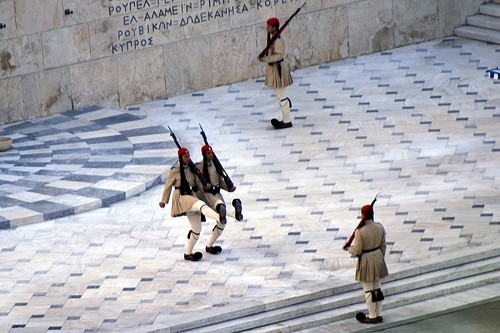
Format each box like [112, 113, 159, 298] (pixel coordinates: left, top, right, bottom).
[198, 123, 234, 191]
[343, 192, 380, 251]
[258, 1, 307, 61]
[167, 124, 208, 187]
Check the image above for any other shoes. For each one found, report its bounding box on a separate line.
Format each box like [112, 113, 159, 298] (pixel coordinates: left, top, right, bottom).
[271, 118, 292, 129]
[232, 199, 243, 221]
[206, 246, 222, 254]
[356, 312, 376, 323]
[375, 315, 383, 322]
[216, 203, 227, 224]
[184, 252, 203, 261]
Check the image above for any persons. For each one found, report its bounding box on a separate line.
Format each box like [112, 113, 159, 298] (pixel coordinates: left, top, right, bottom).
[344, 205, 390, 324]
[257, 17, 293, 130]
[159, 144, 244, 257]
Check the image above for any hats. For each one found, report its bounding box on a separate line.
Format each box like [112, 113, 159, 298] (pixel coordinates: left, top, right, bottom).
[361, 205, 371, 211]
[178, 148, 189, 156]
[267, 18, 280, 25]
[202, 145, 212, 151]
